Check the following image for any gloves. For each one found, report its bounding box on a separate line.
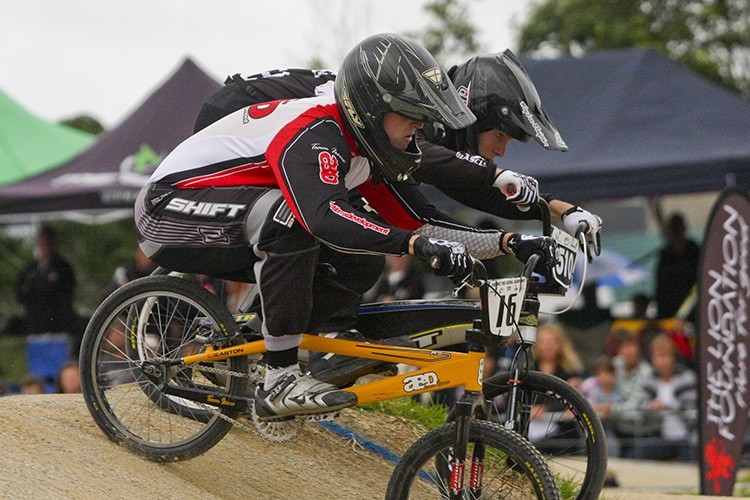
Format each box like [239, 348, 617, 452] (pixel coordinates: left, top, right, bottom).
[561, 205, 603, 264]
[412, 234, 488, 287]
[506, 232, 561, 277]
[491, 168, 541, 212]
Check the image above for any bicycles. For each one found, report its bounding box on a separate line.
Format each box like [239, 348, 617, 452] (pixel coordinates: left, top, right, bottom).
[77, 250, 564, 500]
[126, 184, 608, 500]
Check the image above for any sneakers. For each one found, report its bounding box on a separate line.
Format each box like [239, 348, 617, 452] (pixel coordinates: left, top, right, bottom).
[307, 329, 418, 388]
[252, 371, 357, 423]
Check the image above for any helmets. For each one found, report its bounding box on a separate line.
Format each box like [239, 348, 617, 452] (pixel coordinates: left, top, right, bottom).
[333, 32, 478, 184]
[447, 47, 568, 152]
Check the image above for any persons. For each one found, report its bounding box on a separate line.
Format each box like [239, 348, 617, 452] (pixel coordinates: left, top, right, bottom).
[376, 254, 424, 301]
[452, 211, 697, 456]
[134, 34, 558, 421]
[193, 48, 603, 383]
[17, 229, 254, 393]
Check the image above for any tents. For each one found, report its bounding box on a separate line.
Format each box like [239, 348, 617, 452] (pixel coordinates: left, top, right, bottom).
[0, 58, 221, 219]
[421, 50, 750, 206]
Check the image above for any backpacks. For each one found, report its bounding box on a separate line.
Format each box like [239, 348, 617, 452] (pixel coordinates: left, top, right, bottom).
[194, 67, 342, 133]
